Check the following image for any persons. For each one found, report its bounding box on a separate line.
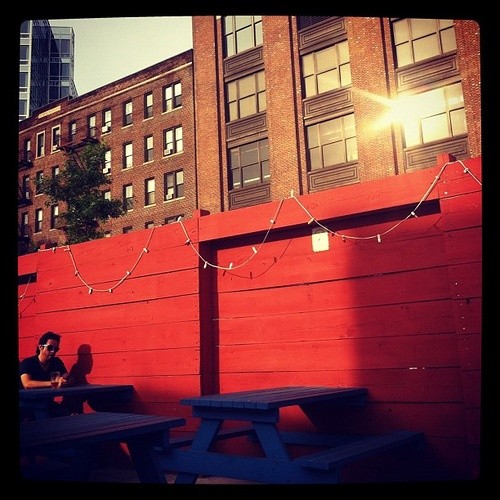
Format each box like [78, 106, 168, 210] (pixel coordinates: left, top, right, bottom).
[18, 331, 74, 424]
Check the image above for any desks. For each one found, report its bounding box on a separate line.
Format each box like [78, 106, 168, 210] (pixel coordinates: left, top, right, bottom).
[19, 384, 368, 485]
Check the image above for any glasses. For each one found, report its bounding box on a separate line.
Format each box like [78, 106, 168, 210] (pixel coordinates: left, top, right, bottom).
[42, 345, 59, 352]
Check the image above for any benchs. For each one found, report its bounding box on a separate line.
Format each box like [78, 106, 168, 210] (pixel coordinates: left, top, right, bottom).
[142, 424, 426, 484]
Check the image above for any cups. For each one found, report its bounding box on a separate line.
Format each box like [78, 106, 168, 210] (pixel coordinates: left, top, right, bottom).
[50, 371, 60, 389]
